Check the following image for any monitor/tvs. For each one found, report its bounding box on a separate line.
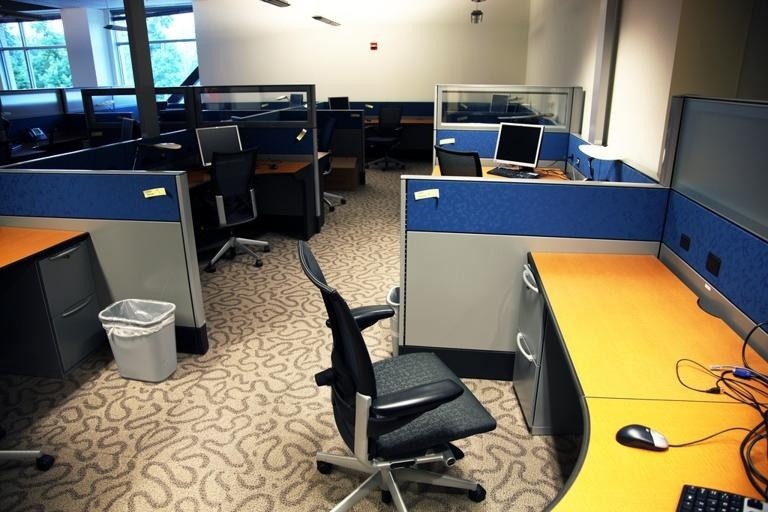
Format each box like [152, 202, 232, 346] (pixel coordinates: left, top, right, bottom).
[490, 93, 510, 112]
[290, 93, 303, 107]
[495, 122, 545, 173]
[328, 97, 351, 110]
[195, 125, 243, 166]
[120, 117, 135, 140]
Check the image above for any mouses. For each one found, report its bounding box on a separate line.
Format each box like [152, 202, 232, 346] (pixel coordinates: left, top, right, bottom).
[616, 424, 669, 452]
[270, 163, 278, 169]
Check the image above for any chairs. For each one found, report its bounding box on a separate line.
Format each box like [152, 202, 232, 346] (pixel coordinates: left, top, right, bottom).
[434, 145, 482, 177]
[319, 152, 345, 212]
[366, 105, 401, 169]
[297, 238, 494, 512]
[0, 449, 54, 471]
[192, 148, 271, 273]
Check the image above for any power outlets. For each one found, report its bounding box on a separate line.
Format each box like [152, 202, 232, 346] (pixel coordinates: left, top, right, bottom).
[570, 154, 574, 162]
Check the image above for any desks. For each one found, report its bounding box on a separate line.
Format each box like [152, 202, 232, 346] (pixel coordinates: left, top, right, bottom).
[431, 163, 570, 180]
[185, 160, 313, 193]
[0, 226, 101, 377]
[511, 252, 768, 512]
[364, 116, 433, 126]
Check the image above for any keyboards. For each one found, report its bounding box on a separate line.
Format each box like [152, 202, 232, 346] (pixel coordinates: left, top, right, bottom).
[487, 167, 540, 179]
[676, 484, 768, 512]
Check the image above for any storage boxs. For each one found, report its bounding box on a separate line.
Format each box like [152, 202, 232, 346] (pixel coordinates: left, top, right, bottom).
[325, 157, 359, 191]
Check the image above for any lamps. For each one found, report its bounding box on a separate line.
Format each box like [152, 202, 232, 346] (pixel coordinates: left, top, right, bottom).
[470, 0, 484, 24]
[578, 144, 624, 178]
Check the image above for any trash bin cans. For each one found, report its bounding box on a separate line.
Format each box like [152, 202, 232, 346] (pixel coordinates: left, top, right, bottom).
[98, 299, 178, 383]
[388, 287, 399, 357]
